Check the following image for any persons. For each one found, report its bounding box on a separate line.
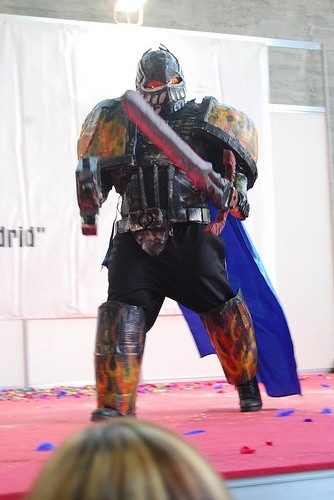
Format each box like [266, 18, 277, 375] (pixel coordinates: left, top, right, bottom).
[75, 45, 259, 418]
[27, 419, 230, 500]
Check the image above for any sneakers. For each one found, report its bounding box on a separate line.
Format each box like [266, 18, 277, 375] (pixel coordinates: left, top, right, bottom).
[91, 406, 121, 421]
[236, 378, 262, 411]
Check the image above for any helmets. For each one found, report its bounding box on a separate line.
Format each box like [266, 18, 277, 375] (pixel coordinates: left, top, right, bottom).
[134, 43, 187, 116]
